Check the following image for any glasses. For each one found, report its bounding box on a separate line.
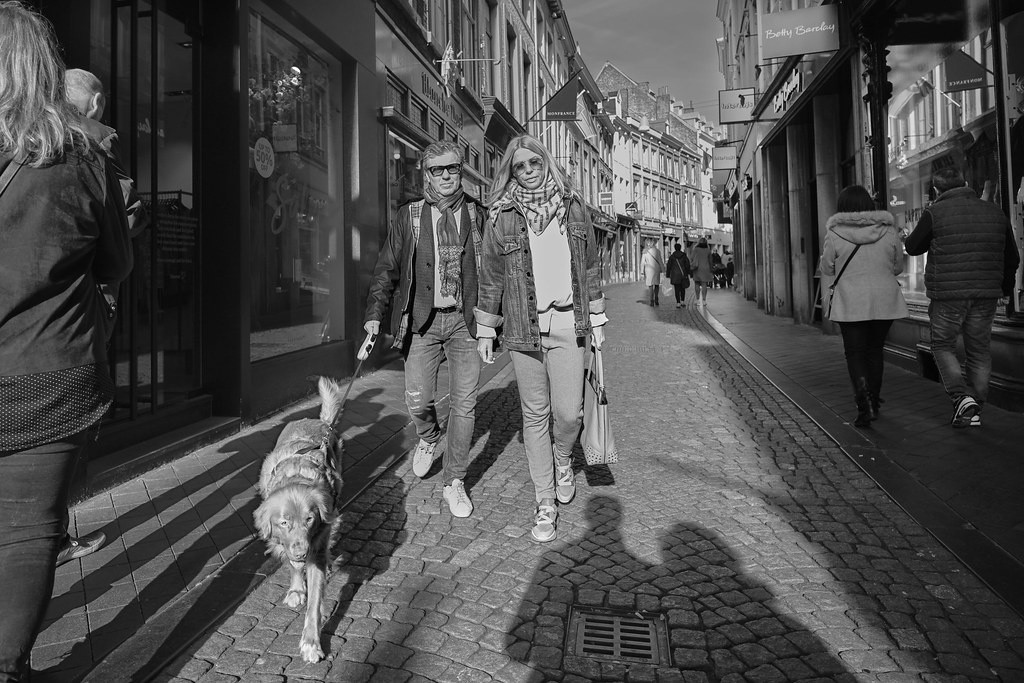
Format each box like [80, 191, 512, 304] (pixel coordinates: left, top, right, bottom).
[427, 162, 462, 176]
[511, 157, 543, 177]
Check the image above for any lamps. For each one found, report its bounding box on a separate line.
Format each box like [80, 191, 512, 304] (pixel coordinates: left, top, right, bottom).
[755, 62, 784, 80]
[432, 58, 501, 65]
[555, 156, 574, 165]
[739, 93, 764, 107]
[720, 140, 744, 147]
[292, 51, 307, 75]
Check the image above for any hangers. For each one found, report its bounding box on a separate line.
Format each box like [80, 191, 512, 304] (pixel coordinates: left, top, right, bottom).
[139, 189, 189, 210]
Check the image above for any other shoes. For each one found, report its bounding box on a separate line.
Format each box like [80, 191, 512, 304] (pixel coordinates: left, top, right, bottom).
[681, 301, 686, 306]
[654, 296, 659, 305]
[696, 299, 700, 305]
[650, 300, 654, 306]
[703, 300, 707, 306]
[676, 302, 681, 308]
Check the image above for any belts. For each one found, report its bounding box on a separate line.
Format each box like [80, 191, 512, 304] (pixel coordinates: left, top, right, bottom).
[432, 305, 457, 314]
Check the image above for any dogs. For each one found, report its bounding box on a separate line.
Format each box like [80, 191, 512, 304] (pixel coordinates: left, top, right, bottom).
[253, 375, 345, 663]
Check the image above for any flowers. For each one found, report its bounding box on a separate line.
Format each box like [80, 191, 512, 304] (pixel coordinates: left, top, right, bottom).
[247, 67, 331, 123]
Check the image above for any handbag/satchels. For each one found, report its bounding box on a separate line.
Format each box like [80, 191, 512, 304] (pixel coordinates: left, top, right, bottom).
[682, 275, 690, 288]
[579, 344, 619, 466]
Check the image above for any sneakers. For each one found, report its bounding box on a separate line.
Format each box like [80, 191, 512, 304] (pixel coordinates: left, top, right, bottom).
[443, 477, 473, 518]
[532, 498, 557, 542]
[969, 412, 981, 426]
[550, 443, 576, 503]
[56, 530, 106, 565]
[411, 439, 436, 478]
[952, 396, 975, 428]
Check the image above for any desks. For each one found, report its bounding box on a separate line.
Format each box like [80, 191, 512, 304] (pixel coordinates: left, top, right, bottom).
[810, 276, 820, 323]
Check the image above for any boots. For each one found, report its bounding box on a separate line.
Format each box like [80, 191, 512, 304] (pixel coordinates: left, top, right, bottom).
[866, 374, 881, 420]
[853, 377, 872, 426]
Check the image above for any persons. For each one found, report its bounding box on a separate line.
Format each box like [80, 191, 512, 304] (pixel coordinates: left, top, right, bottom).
[1011, 113, 1024, 204]
[709, 248, 735, 288]
[55, 69, 147, 567]
[641, 239, 666, 307]
[819, 185, 909, 425]
[473, 131, 609, 542]
[364, 138, 503, 518]
[691, 237, 713, 306]
[905, 167, 1020, 428]
[666, 243, 691, 307]
[964, 127, 997, 203]
[0, 3, 135, 683]
